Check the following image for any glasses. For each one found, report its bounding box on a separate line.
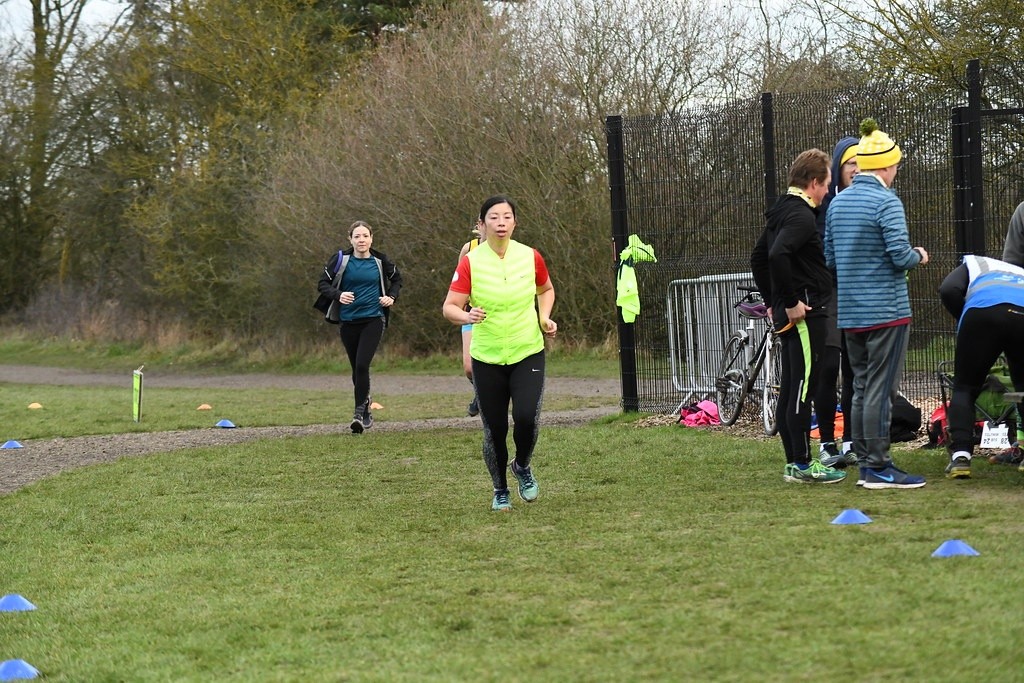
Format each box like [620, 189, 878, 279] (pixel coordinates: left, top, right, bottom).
[845, 161, 858, 171]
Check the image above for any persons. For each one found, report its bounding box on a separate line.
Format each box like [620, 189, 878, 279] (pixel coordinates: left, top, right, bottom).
[457, 212, 487, 416]
[938, 255, 1024, 478]
[822, 118, 929, 489]
[443, 197, 557, 510]
[813, 137, 857, 467]
[750, 148, 846, 484]
[314, 221, 403, 434]
[1003, 201, 1024, 266]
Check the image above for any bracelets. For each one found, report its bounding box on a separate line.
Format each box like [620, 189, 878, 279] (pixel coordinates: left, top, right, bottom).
[390, 295, 395, 300]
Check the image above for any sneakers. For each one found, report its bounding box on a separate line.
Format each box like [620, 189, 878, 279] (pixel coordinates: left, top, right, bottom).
[863, 461, 927, 489]
[790, 457, 847, 483]
[363, 394, 373, 428]
[783, 462, 795, 483]
[856, 466, 866, 486]
[990, 442, 1024, 466]
[840, 448, 858, 466]
[491, 487, 513, 511]
[350, 414, 364, 433]
[944, 455, 973, 478]
[509, 456, 539, 501]
[818, 442, 848, 468]
[467, 396, 479, 416]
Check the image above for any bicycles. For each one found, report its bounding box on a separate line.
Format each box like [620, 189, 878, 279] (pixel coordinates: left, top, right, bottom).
[712, 285, 783, 437]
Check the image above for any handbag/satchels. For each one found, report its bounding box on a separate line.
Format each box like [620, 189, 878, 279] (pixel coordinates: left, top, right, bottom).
[921, 398, 986, 449]
[677, 400, 722, 428]
[886, 395, 922, 443]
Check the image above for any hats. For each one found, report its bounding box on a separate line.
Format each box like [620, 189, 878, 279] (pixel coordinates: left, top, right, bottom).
[856, 116, 903, 170]
[839, 145, 859, 168]
